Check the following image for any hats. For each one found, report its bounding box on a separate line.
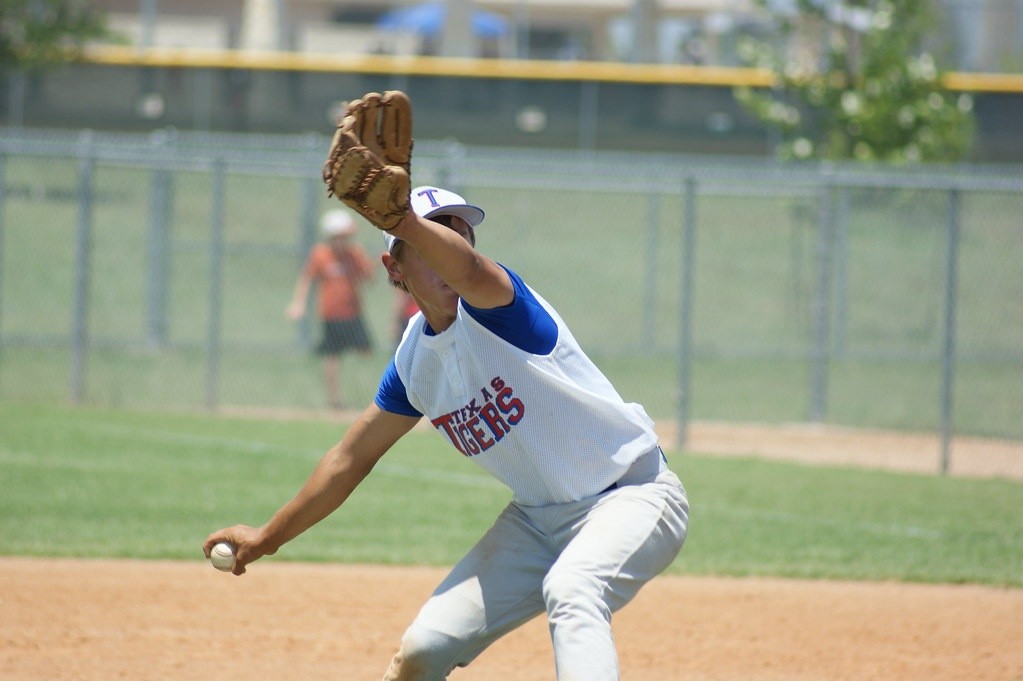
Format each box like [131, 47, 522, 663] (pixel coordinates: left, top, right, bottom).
[382, 186, 485, 252]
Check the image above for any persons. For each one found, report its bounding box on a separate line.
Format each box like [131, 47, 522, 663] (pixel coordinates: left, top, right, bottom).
[202, 89, 690, 681]
[280, 210, 421, 409]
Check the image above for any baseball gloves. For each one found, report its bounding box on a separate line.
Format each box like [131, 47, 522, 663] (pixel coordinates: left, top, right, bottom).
[323, 89, 414, 232]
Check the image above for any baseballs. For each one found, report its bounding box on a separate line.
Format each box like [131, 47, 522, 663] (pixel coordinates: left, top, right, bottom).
[211, 542, 236, 573]
